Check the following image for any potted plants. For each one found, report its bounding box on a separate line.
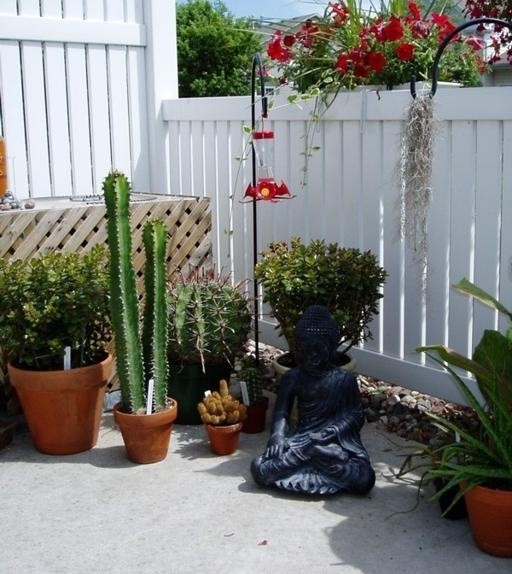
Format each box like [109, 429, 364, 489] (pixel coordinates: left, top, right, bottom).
[387, 277, 512, 562]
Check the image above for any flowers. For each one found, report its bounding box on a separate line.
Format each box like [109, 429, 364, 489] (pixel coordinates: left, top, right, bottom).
[222, 0, 495, 239]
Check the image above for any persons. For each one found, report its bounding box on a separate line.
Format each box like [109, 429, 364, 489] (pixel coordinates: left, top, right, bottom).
[250, 304, 374, 501]
